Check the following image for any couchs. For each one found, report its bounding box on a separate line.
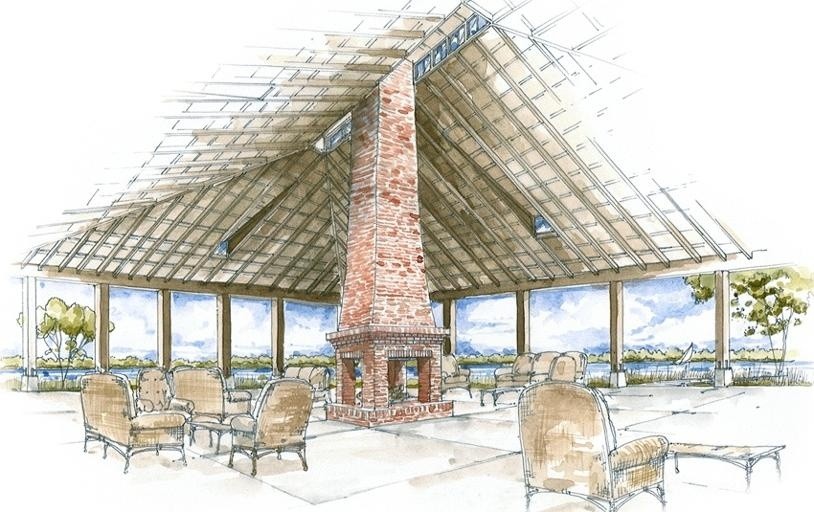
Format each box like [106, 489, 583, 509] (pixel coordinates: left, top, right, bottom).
[493, 350, 588, 387]
[284, 365, 332, 409]
[79, 364, 251, 473]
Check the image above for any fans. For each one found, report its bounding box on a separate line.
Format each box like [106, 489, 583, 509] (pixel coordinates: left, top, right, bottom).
[666, 442, 786, 485]
[478, 385, 526, 406]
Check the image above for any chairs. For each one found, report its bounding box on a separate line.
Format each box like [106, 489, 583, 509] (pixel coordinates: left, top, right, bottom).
[440, 353, 472, 398]
[543, 356, 576, 382]
[227, 377, 316, 476]
[518, 381, 668, 512]
